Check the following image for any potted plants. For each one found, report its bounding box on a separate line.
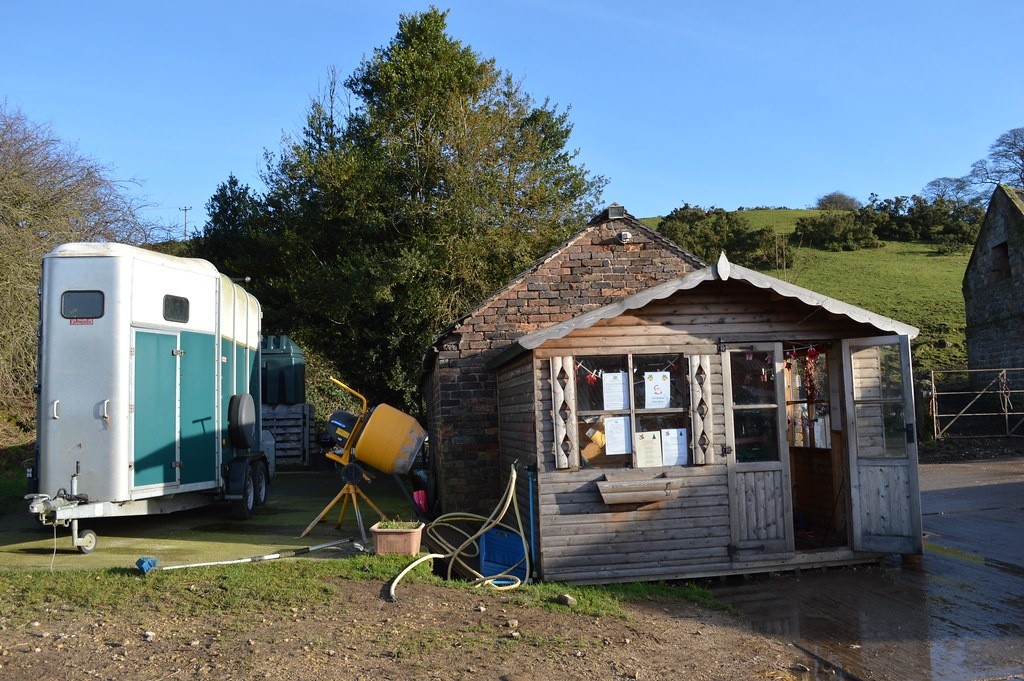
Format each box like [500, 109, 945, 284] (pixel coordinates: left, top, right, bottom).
[369, 515, 425, 557]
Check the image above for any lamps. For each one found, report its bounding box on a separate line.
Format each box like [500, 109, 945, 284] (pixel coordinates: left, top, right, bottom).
[608, 205, 625, 219]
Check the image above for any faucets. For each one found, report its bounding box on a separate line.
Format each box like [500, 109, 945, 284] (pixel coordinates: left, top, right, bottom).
[512, 455, 520, 469]
